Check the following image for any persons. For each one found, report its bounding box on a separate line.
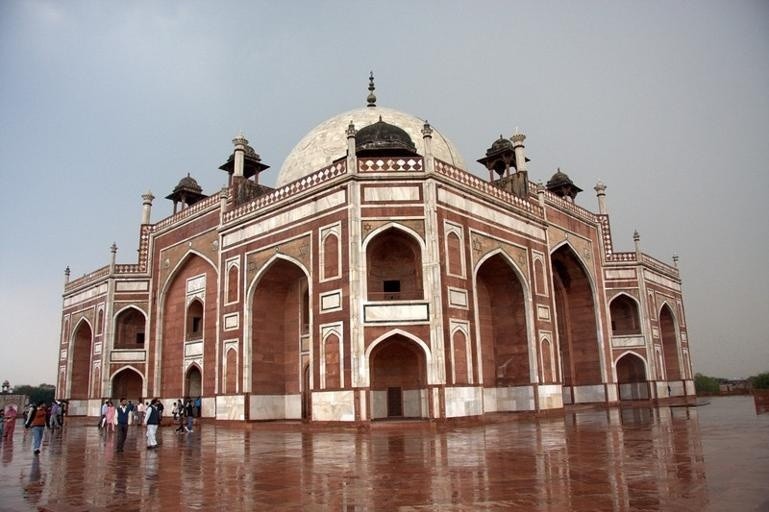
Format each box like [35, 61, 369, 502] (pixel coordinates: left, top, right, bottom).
[0, 399, 69, 454]
[98, 396, 200, 454]
